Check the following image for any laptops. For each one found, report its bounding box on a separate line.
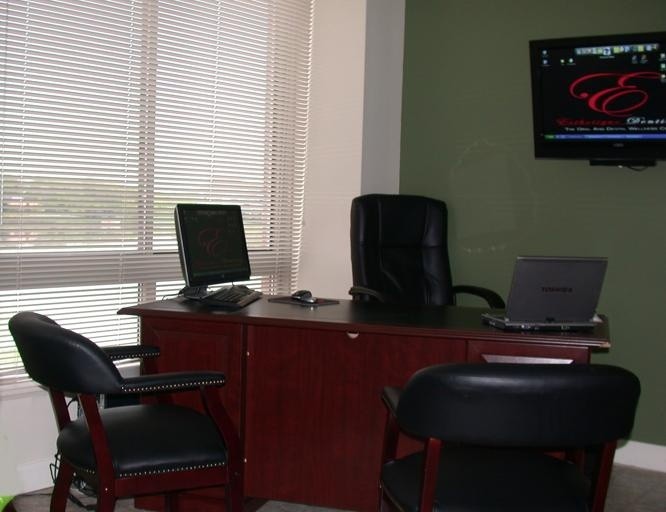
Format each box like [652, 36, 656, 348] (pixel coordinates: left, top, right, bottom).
[480, 256, 609, 331]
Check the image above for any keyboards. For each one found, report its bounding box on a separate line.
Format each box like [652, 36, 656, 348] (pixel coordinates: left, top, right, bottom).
[202, 285, 263, 307]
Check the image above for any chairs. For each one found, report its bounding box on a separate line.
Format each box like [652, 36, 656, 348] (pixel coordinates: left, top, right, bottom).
[9, 312, 244, 512]
[378, 362, 642, 512]
[349, 194, 505, 309]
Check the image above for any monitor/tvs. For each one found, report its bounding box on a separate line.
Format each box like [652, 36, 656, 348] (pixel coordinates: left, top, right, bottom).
[529, 31, 666, 166]
[174, 203, 252, 300]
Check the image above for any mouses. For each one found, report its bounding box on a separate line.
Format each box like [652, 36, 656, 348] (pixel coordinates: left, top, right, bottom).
[292, 290, 318, 302]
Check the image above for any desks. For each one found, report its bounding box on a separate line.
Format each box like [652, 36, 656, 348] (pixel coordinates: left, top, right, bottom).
[118, 291, 614, 511]
